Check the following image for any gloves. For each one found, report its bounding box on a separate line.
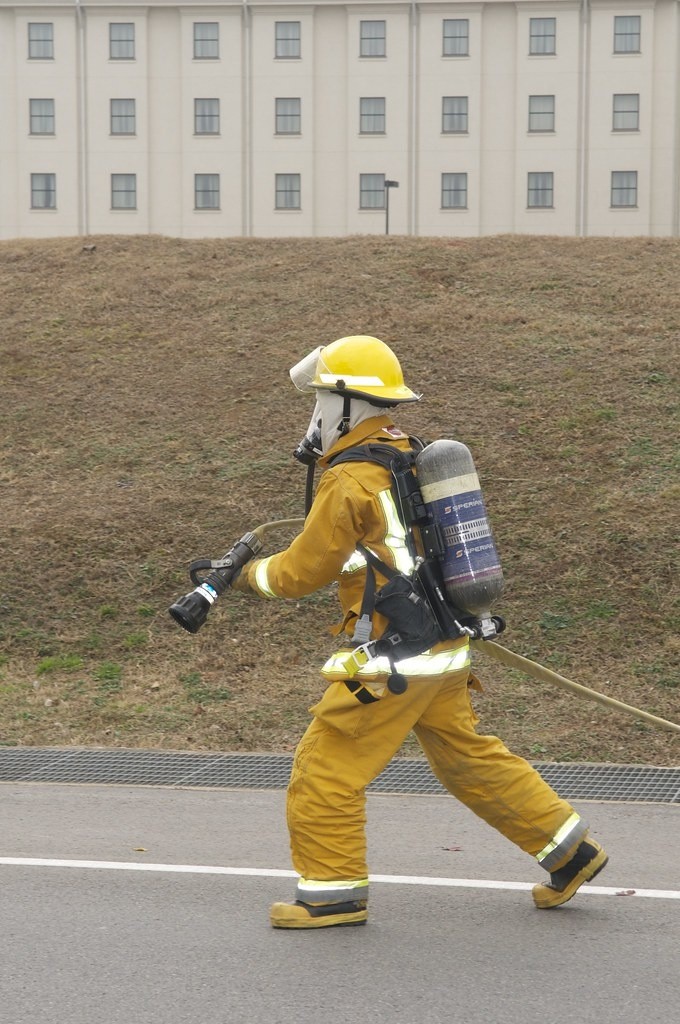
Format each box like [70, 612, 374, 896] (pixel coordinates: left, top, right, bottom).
[231, 558, 257, 594]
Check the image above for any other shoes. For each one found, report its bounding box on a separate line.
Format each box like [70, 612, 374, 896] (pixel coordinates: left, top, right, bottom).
[531, 836, 610, 909]
[268, 900, 368, 929]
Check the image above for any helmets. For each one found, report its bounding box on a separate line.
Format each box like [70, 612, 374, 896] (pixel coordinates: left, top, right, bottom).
[308, 336, 419, 408]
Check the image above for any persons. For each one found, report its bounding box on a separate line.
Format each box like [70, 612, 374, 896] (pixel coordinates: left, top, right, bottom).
[230, 334, 609, 930]
